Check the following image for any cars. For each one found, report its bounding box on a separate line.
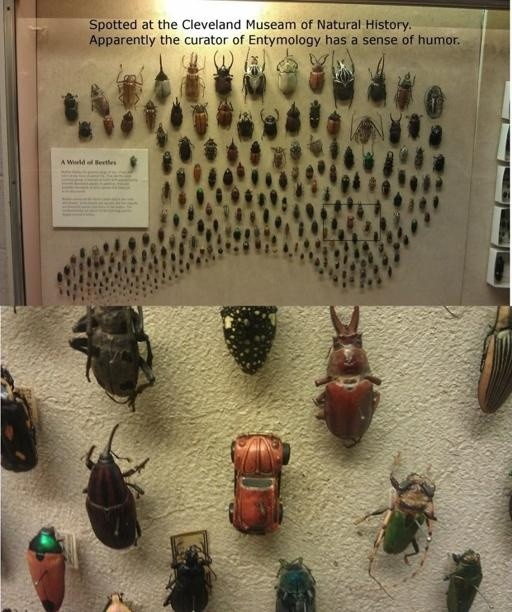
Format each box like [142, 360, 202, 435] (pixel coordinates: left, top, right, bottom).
[227, 434, 291, 536]
